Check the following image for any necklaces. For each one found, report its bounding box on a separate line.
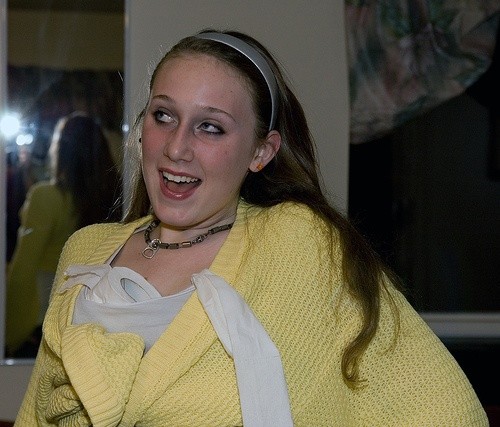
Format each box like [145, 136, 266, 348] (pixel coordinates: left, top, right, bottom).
[142, 219, 235, 260]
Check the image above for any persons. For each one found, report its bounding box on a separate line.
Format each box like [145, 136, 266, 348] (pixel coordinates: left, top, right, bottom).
[4, 111, 124, 361]
[6, 122, 50, 263]
[15, 30, 493, 427]
